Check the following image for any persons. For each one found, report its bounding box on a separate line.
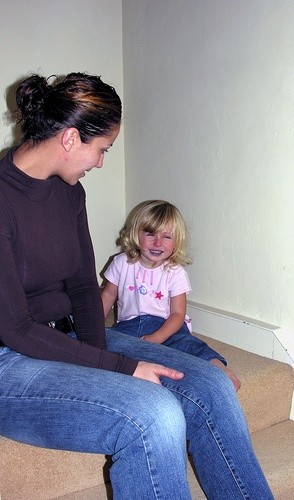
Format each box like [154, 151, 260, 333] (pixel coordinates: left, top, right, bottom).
[0, 70, 276, 500]
[101, 199, 242, 394]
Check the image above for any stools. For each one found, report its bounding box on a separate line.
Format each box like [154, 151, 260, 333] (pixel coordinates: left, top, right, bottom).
[0, 307, 294, 500]
